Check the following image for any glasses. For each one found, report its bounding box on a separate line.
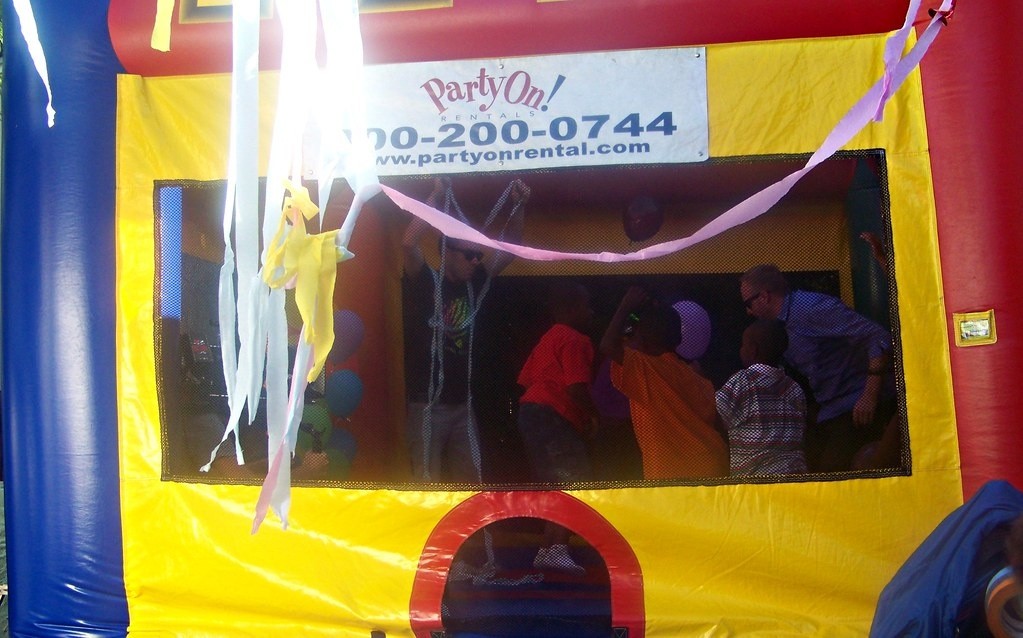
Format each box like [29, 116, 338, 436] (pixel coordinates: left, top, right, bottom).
[744, 290, 763, 309]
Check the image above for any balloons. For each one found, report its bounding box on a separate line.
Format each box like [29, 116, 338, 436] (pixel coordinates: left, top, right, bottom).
[319, 309, 365, 468]
[623, 195, 664, 243]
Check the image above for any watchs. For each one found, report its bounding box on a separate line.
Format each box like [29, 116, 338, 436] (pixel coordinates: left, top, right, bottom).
[867, 366, 889, 376]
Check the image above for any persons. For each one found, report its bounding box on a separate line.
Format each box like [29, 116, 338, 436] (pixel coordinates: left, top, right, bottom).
[399, 176, 890, 489]
[161, 356, 328, 482]
